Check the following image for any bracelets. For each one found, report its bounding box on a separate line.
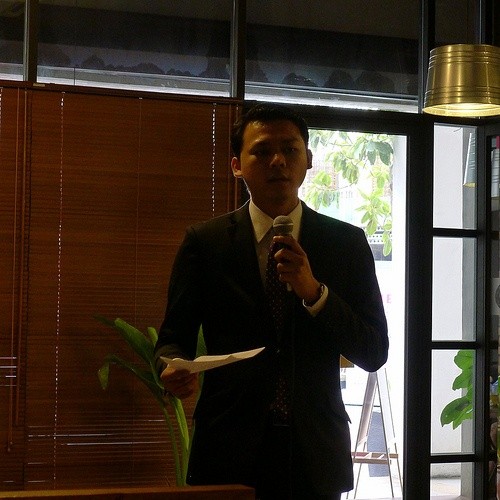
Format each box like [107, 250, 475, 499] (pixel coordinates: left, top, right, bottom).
[305, 281, 324, 308]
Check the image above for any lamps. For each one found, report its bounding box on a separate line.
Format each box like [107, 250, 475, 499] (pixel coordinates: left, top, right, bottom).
[421, 1, 500, 119]
[490, 147, 500, 200]
[464, 132, 476, 188]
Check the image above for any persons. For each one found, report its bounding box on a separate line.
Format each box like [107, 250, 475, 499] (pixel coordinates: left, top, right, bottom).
[151, 101, 390, 500]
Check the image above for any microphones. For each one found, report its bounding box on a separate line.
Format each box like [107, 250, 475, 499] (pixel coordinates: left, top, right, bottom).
[273, 215, 294, 303]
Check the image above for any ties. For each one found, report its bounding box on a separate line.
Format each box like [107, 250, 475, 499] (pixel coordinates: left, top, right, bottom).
[265, 236, 289, 299]
